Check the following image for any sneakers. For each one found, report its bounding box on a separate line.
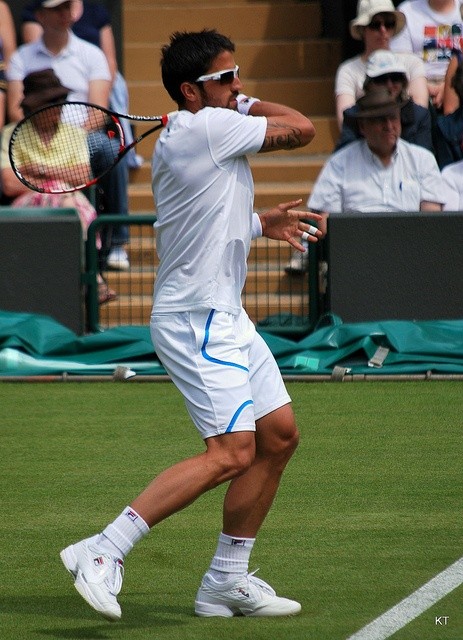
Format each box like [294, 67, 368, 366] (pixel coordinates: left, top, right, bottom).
[286, 240, 312, 276]
[194, 568, 303, 620]
[59, 535, 127, 623]
[107, 245, 133, 273]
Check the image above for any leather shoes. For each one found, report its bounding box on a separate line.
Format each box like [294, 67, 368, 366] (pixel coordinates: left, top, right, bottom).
[94, 275, 117, 304]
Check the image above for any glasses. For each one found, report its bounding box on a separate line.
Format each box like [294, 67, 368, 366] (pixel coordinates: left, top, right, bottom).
[376, 74, 406, 86]
[42, 93, 69, 104]
[367, 19, 398, 33]
[196, 65, 241, 85]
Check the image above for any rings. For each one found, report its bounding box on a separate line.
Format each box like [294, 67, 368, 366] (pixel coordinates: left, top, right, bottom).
[301, 232, 309, 240]
[307, 226, 317, 235]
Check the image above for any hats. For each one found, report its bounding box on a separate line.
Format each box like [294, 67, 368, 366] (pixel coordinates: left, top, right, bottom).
[19, 69, 73, 107]
[351, 0, 407, 39]
[32, 0, 74, 10]
[343, 90, 409, 131]
[365, 49, 411, 94]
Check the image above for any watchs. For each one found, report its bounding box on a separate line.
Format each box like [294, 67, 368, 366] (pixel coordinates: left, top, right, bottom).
[37, 165, 45, 178]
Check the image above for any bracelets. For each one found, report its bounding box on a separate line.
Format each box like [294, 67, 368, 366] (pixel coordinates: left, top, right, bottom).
[250, 212, 262, 240]
[235, 94, 260, 116]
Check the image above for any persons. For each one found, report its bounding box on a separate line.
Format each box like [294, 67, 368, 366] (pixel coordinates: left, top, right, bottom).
[441, 132, 463, 212]
[0, 69, 116, 304]
[18, 1, 130, 167]
[388, 0, 462, 109]
[5, 0, 132, 271]
[283, 50, 431, 277]
[307, 88, 446, 237]
[442, 2, 462, 115]
[333, 0, 429, 133]
[57, 27, 322, 623]
[436, 59, 462, 163]
[0, 0, 17, 130]
